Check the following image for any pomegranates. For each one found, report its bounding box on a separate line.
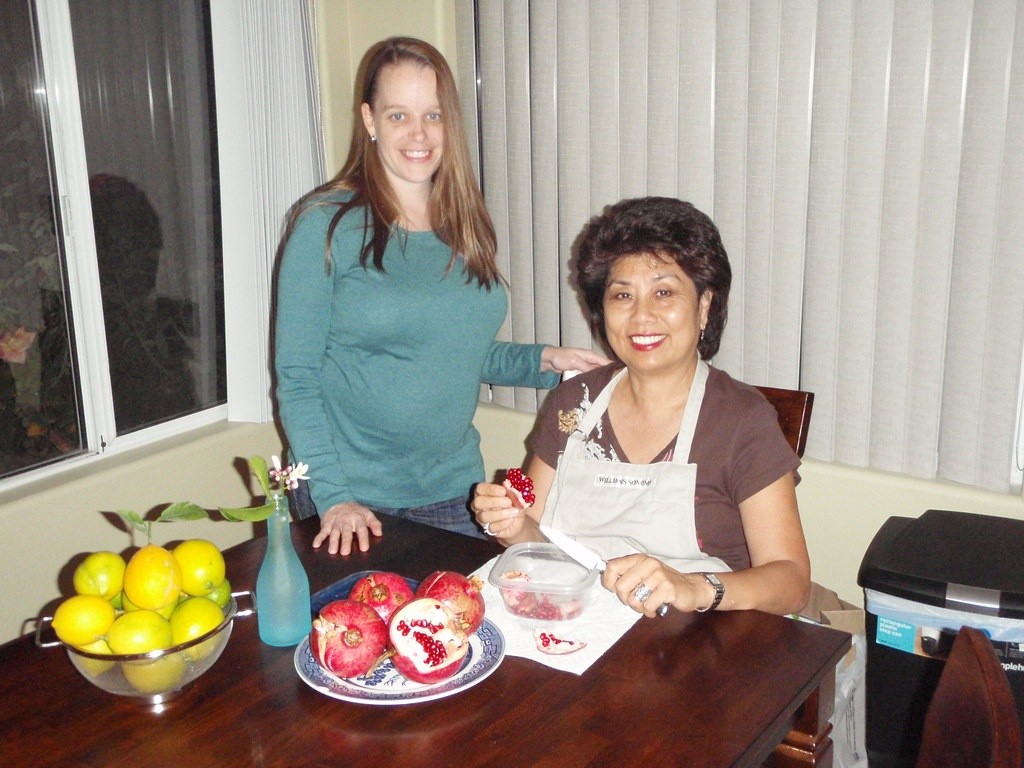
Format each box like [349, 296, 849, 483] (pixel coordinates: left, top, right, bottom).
[503, 468, 536, 510]
[508, 575, 562, 620]
[533, 628, 587, 655]
[311, 570, 486, 685]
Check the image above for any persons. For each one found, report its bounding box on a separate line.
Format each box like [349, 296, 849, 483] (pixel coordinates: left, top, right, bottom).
[275, 38, 611, 556]
[470, 197, 811, 614]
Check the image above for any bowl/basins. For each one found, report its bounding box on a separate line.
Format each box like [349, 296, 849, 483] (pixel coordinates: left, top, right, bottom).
[487, 542, 600, 620]
[36, 588, 257, 703]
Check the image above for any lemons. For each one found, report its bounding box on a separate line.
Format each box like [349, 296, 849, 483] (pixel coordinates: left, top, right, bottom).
[51, 539, 233, 692]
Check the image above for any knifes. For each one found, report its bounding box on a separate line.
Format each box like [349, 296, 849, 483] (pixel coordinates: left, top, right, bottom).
[539, 524, 668, 617]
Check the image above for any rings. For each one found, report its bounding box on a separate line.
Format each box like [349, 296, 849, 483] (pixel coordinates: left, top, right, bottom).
[483, 524, 497, 536]
[634, 584, 652, 602]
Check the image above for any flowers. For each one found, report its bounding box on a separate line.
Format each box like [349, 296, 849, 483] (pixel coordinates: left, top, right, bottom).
[0, 325, 36, 365]
[219, 457, 310, 522]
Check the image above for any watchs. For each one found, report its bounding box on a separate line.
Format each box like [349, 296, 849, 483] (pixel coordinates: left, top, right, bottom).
[691, 572, 725, 614]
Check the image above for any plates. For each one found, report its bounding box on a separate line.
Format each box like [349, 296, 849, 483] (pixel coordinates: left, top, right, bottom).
[310, 571, 421, 615]
[294, 616, 506, 706]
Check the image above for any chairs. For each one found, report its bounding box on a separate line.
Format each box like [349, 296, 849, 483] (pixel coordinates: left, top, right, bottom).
[755, 386, 816, 458]
[914, 625, 1024, 768]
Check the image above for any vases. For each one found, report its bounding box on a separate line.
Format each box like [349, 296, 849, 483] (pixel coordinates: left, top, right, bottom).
[255, 497, 314, 648]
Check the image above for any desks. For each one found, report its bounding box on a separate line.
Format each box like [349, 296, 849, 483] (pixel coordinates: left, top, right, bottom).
[0, 509, 854, 768]
